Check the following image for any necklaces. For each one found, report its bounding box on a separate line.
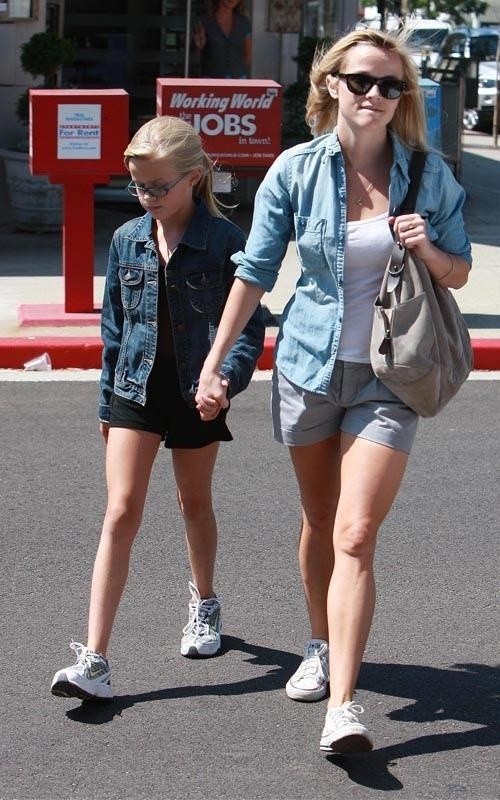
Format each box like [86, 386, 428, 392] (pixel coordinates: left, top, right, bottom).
[352, 160, 388, 207]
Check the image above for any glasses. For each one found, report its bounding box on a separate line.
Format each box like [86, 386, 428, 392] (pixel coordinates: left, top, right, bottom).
[330, 70, 407, 100]
[128, 171, 189, 197]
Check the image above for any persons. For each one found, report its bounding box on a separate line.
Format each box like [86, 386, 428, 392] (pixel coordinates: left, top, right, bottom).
[48, 112, 265, 700]
[188, 0, 253, 80]
[186, 27, 474, 756]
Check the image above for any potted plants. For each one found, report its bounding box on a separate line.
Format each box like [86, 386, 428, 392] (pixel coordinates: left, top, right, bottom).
[3, 32, 85, 234]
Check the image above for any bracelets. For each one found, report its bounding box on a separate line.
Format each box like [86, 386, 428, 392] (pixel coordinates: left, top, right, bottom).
[433, 254, 454, 284]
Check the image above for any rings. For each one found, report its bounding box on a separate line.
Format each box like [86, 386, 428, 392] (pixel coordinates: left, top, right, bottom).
[408, 220, 417, 229]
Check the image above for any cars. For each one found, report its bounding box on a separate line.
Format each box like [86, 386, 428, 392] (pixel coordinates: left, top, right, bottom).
[362, 6, 500, 132]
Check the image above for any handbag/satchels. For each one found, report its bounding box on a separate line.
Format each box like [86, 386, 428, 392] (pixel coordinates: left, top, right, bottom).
[369, 242, 473, 418]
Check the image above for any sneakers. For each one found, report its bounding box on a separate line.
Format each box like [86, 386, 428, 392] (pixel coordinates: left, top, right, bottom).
[180, 580, 222, 656]
[319, 700, 374, 752]
[51, 638, 114, 704]
[285, 639, 329, 703]
